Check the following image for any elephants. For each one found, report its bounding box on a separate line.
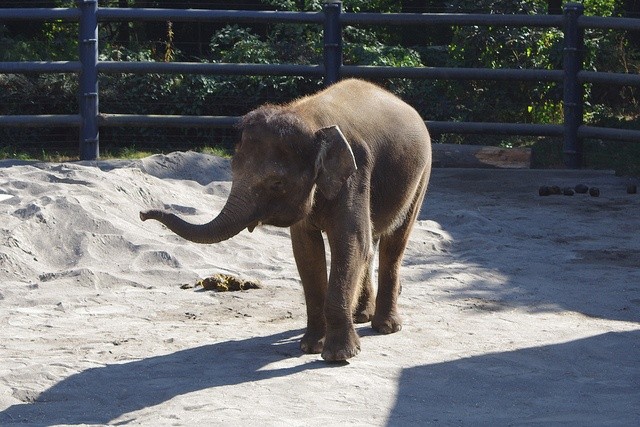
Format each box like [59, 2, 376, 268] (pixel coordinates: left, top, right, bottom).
[140, 78, 432, 364]
